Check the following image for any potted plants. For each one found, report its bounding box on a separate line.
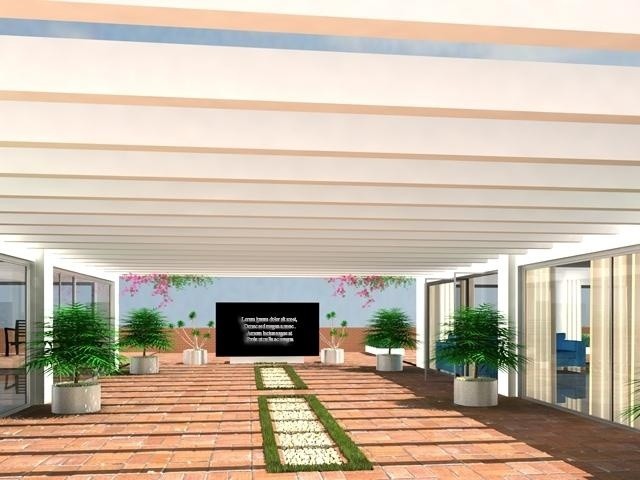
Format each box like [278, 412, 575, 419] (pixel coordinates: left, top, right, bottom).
[169, 312, 213, 371]
[26, 300, 126, 415]
[434, 302, 532, 407]
[319, 310, 348, 365]
[365, 306, 417, 372]
[119, 306, 172, 375]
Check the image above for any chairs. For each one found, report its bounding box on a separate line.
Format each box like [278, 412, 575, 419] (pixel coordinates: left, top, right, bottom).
[4, 319, 26, 356]
[4, 374, 25, 394]
